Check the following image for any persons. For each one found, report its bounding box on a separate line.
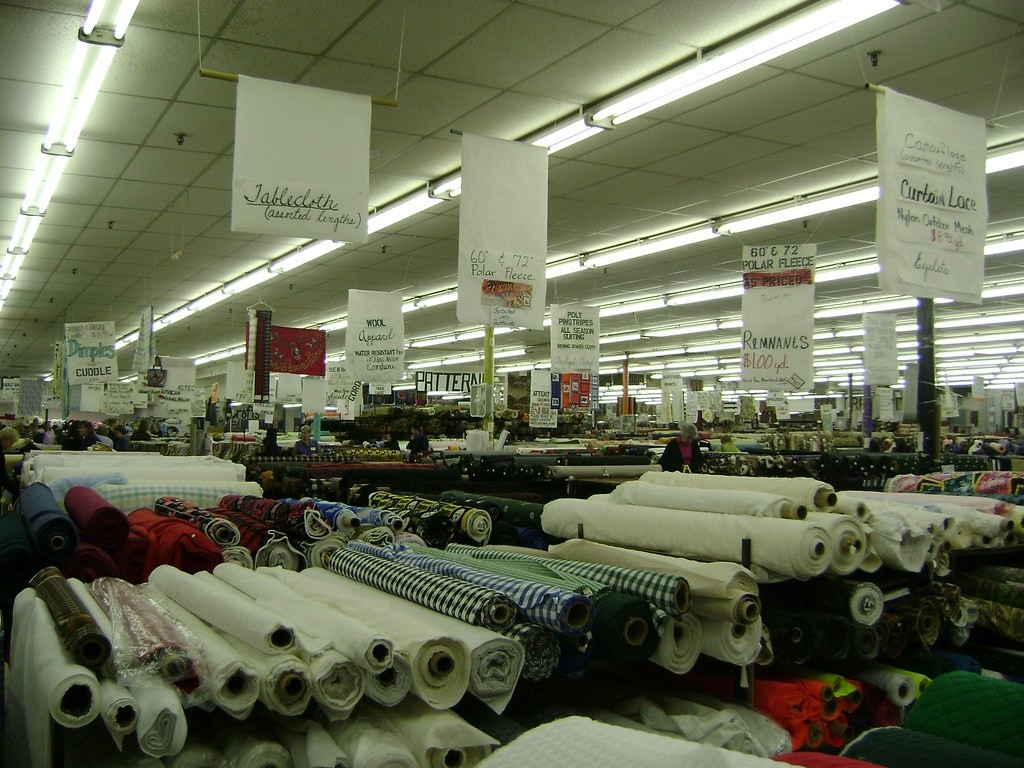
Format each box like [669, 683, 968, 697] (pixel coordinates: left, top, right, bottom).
[381, 431, 400, 450]
[295, 425, 323, 455]
[716, 435, 740, 452]
[261, 426, 280, 455]
[657, 421, 704, 474]
[0, 417, 178, 515]
[406, 424, 429, 463]
[953, 425, 1024, 438]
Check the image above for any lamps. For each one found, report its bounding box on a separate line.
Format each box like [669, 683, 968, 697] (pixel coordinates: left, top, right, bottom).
[0, 0, 1024, 411]
